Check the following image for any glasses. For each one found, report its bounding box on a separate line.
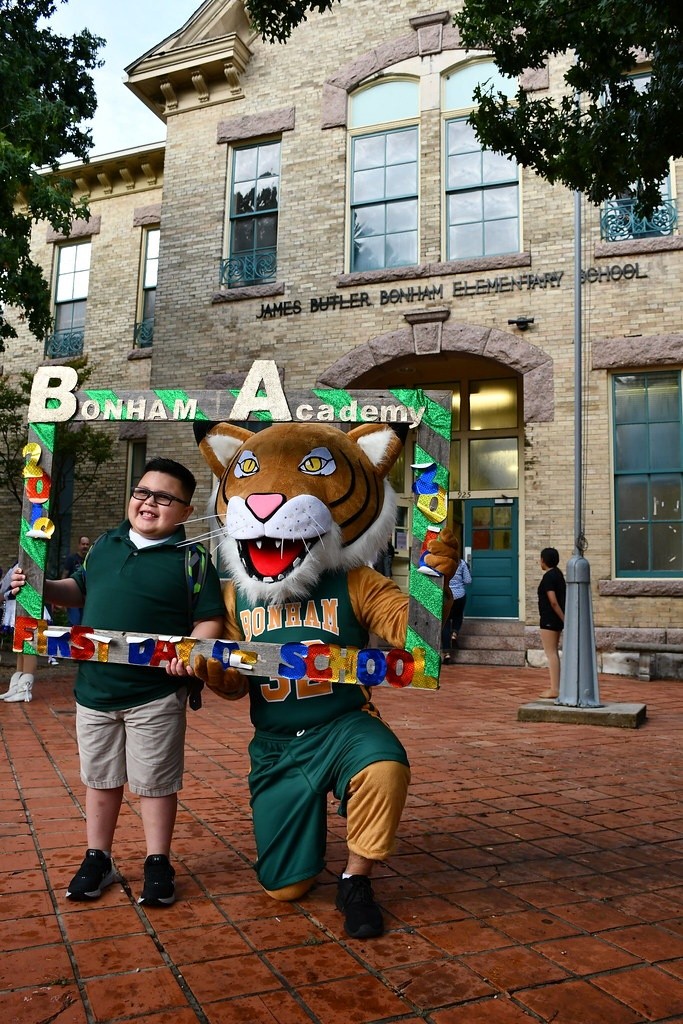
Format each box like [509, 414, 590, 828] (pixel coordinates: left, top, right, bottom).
[130, 485, 190, 506]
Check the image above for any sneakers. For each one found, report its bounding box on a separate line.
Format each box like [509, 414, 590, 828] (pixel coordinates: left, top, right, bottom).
[335, 867, 385, 938]
[65, 849, 117, 902]
[136, 854, 175, 907]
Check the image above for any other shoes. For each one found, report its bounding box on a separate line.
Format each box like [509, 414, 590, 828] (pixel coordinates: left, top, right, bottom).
[444, 654, 453, 664]
[539, 690, 559, 697]
[47, 658, 59, 664]
[452, 631, 459, 642]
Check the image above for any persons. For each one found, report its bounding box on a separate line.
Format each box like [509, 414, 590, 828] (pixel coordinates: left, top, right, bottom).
[0, 536, 89, 703]
[440, 548, 566, 698]
[373, 542, 394, 579]
[11, 457, 229, 907]
[193, 421, 463, 938]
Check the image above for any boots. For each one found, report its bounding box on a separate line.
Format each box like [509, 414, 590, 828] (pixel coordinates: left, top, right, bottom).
[3, 673, 34, 702]
[0, 671, 24, 699]
